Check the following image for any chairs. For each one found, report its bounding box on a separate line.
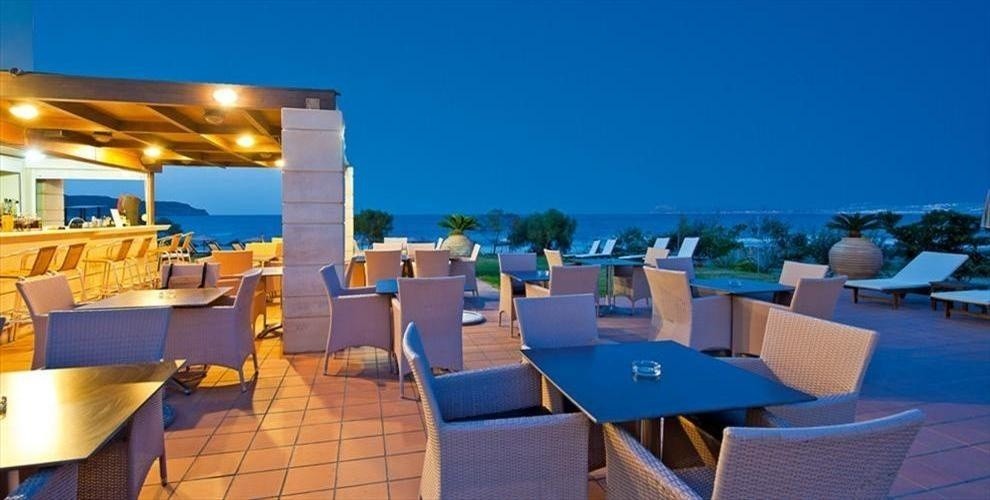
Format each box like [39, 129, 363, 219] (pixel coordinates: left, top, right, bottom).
[843, 251, 969, 310]
[929, 289, 990, 320]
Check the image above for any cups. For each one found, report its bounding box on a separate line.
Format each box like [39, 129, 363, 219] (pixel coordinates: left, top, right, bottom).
[71, 223, 82, 228]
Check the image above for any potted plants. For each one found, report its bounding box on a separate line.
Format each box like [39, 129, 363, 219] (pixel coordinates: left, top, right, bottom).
[823, 212, 891, 280]
[437, 212, 482, 257]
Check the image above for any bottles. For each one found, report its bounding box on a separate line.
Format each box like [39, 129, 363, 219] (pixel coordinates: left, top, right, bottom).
[3, 198, 42, 232]
[82, 214, 111, 228]
